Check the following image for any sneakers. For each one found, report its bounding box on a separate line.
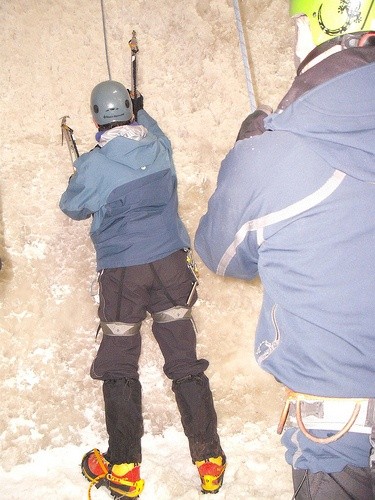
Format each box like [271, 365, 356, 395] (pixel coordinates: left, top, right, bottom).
[80, 448, 144, 500]
[196, 450, 226, 494]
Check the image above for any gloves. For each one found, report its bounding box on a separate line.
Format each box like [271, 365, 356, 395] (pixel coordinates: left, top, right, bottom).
[127, 88, 143, 119]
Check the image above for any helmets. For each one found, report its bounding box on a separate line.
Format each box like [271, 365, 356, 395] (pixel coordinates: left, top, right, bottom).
[91, 80, 133, 125]
[289, 0, 375, 46]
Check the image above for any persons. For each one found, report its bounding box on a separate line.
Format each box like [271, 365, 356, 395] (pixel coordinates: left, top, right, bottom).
[59, 80, 227, 500]
[193, 0, 375, 500]
